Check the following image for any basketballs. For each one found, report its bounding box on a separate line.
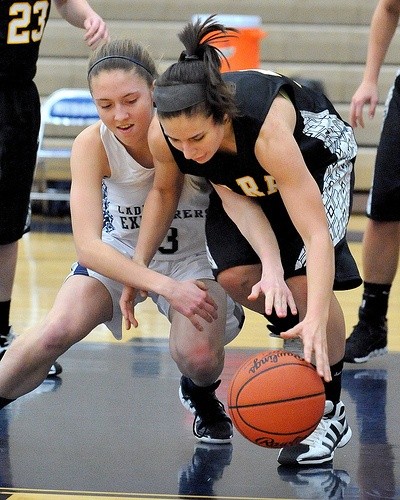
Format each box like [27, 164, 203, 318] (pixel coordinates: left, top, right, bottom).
[229, 350, 325, 449]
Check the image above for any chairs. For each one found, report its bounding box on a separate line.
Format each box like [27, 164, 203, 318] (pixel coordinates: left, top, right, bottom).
[26, 88, 101, 230]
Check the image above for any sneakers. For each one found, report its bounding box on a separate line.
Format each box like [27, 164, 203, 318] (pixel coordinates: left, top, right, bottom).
[276, 400, 352, 465]
[343, 319, 389, 364]
[178, 374, 235, 444]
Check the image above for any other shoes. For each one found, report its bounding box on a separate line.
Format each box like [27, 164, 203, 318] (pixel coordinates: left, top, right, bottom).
[0, 343, 62, 375]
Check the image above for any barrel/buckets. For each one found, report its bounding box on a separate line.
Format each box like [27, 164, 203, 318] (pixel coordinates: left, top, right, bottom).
[191, 13, 265, 71]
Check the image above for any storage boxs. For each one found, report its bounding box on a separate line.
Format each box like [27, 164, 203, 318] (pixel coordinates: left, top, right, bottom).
[200, 28, 264, 72]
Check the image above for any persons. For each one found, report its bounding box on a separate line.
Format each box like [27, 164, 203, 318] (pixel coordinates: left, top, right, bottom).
[0, 37, 236, 444]
[344, 0, 400, 365]
[120, 13, 366, 468]
[0, 1, 111, 379]
